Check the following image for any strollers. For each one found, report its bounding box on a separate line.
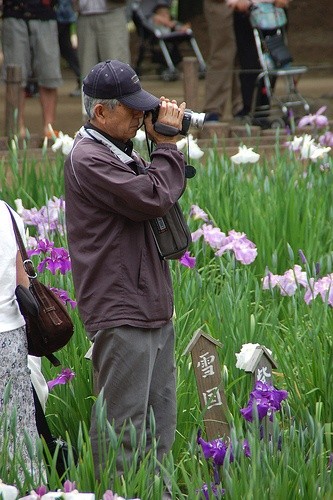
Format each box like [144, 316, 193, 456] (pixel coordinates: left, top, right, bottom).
[133, 0, 209, 83]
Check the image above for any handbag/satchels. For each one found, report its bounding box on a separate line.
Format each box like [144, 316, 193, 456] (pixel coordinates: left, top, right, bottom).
[6, 205, 74, 366]
[146, 199, 192, 261]
[264, 34, 294, 68]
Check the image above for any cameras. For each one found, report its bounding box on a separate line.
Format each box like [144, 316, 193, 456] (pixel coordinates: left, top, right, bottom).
[151, 104, 206, 136]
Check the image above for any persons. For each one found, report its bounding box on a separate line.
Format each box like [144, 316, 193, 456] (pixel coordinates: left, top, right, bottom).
[0, 200, 46, 487]
[24, 0, 82, 97]
[2, 0, 63, 144]
[63, 60, 186, 500]
[229, 0, 287, 117]
[73, 0, 130, 101]
[151, 6, 191, 34]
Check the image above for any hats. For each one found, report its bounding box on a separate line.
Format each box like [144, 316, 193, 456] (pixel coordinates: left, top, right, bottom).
[83, 59, 161, 111]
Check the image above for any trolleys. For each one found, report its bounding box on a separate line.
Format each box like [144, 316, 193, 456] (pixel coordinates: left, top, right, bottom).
[238, 0, 309, 137]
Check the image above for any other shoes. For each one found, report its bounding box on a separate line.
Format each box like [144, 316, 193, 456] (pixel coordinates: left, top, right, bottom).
[204, 114, 221, 125]
[253, 116, 272, 129]
[233, 108, 249, 117]
[24, 82, 40, 98]
[43, 128, 59, 139]
[70, 81, 81, 97]
